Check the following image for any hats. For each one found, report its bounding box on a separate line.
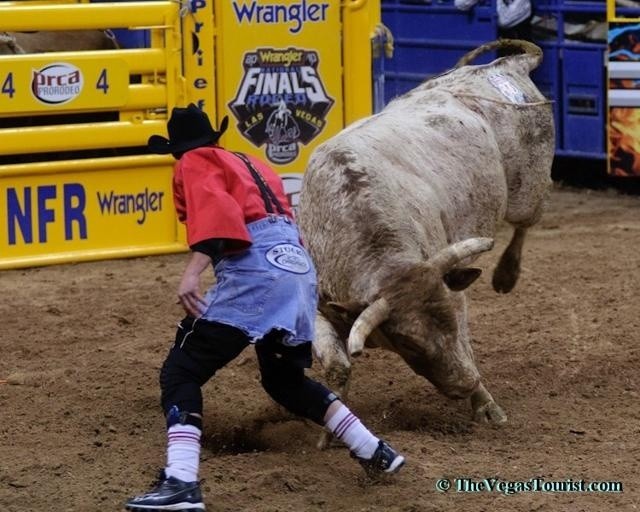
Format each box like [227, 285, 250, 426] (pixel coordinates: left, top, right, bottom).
[144, 102, 229, 157]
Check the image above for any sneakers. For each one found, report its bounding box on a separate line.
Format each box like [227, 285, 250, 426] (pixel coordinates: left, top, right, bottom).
[122, 469, 208, 512]
[348, 438, 405, 481]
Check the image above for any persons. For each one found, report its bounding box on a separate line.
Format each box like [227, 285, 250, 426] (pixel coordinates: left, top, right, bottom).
[126, 103, 406, 512]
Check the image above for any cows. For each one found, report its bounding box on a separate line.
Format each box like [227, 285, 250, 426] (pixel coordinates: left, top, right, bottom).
[0, 28, 137, 166]
[296, 38, 559, 454]
